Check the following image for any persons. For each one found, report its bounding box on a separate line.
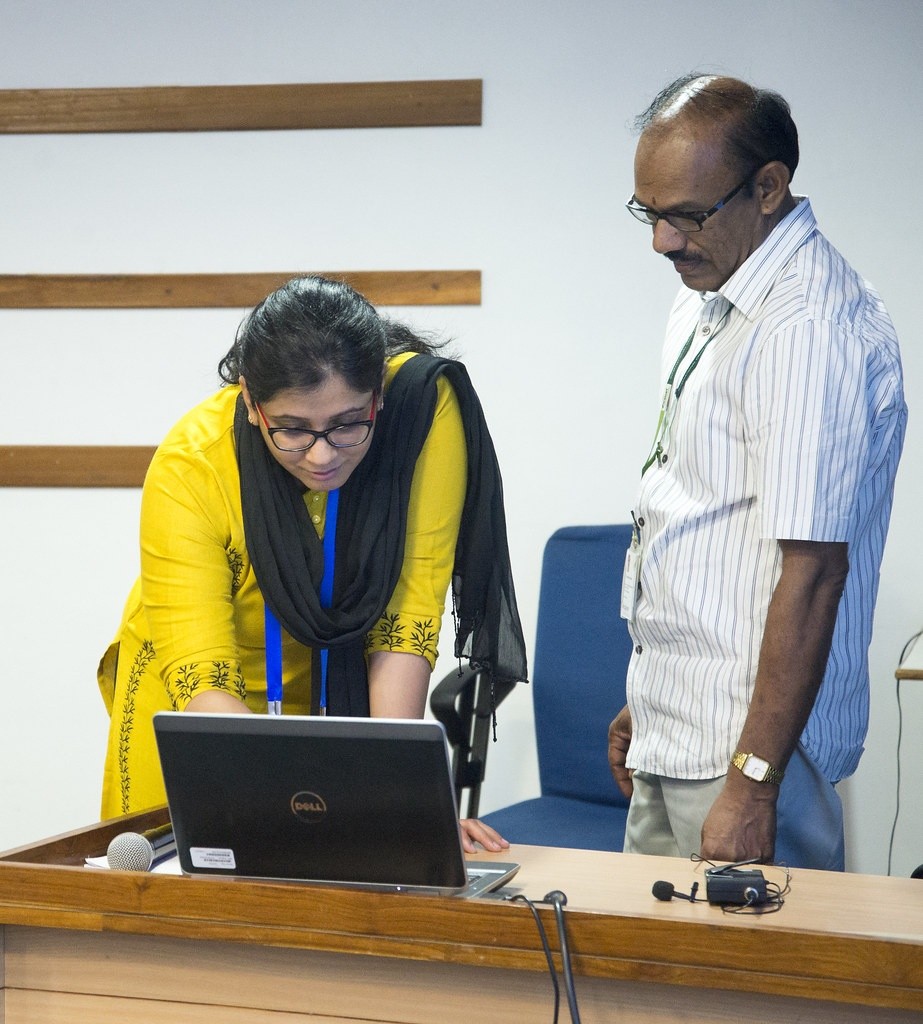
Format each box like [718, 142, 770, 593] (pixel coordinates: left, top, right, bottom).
[610, 72, 910, 865]
[103, 276, 506, 853]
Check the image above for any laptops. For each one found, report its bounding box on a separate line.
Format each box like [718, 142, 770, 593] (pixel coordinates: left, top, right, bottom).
[153, 712, 521, 902]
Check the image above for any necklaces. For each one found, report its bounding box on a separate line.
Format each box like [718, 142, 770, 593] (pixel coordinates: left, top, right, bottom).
[266, 488, 339, 717]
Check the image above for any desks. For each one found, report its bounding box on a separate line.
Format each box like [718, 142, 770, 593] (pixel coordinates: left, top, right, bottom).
[0, 807, 923, 1024]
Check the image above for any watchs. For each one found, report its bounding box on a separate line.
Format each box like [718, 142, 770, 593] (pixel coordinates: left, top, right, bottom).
[730, 750, 785, 786]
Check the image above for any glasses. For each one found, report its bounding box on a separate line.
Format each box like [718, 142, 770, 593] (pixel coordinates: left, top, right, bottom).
[627, 177, 752, 235]
[256, 393, 378, 452]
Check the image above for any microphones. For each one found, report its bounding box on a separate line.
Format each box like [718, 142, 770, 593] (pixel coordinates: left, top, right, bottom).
[652, 880, 675, 902]
[107, 822, 177, 873]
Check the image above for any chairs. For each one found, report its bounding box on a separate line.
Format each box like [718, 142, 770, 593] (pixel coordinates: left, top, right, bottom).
[429, 524, 635, 851]
